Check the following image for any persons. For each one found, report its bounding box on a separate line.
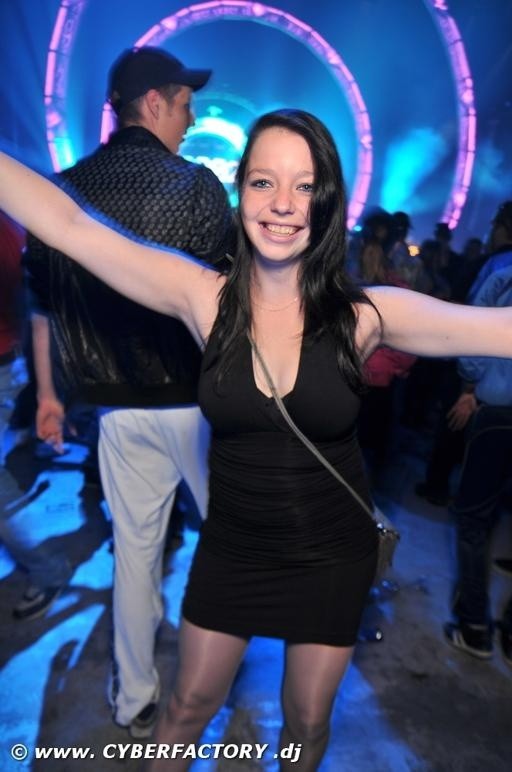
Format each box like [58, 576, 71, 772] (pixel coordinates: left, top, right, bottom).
[0, 47, 512, 772]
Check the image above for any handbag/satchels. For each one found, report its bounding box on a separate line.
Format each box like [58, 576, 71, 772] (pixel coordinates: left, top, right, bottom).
[370, 504, 401, 578]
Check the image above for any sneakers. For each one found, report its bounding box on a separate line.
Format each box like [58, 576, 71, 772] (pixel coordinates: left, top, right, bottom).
[10, 585, 61, 623]
[491, 558, 512, 578]
[109, 662, 158, 739]
[443, 622, 495, 660]
[499, 632, 512, 665]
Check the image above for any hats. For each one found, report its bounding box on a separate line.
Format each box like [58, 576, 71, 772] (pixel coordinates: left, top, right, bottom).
[105, 45, 211, 109]
